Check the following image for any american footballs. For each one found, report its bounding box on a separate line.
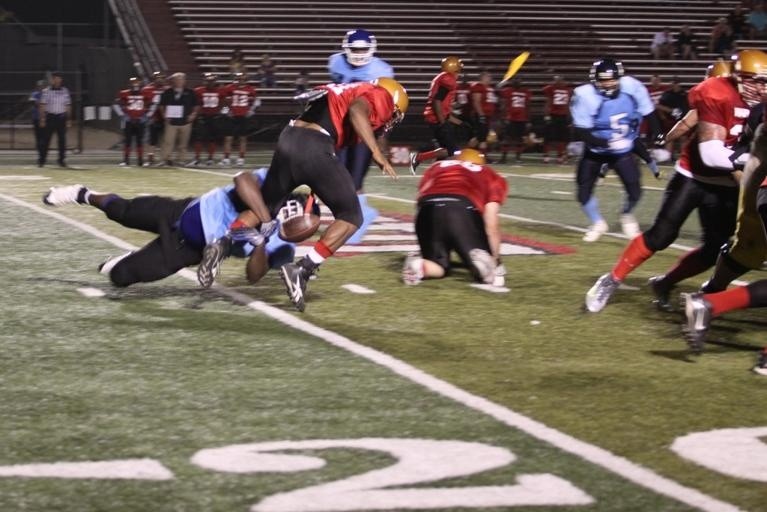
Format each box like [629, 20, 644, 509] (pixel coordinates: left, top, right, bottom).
[278, 213, 320, 242]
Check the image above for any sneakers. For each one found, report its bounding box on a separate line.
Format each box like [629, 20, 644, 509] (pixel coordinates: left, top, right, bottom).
[119, 158, 245, 168]
[679, 291, 714, 353]
[585, 272, 618, 313]
[403, 253, 423, 285]
[648, 275, 676, 313]
[44, 184, 90, 207]
[198, 240, 224, 289]
[468, 248, 505, 287]
[619, 214, 638, 237]
[410, 152, 419, 174]
[279, 263, 307, 312]
[583, 220, 610, 242]
[97, 251, 133, 275]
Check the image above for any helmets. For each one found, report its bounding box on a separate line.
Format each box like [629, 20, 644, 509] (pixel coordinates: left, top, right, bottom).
[371, 77, 409, 133]
[341, 29, 377, 67]
[441, 56, 464, 73]
[276, 193, 322, 242]
[455, 149, 487, 164]
[591, 60, 625, 96]
[706, 49, 766, 108]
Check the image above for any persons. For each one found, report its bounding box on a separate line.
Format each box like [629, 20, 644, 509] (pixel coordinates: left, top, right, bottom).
[586, 49, 767, 313]
[680, 176, 767, 376]
[698, 119, 767, 295]
[26, 76, 45, 154]
[42, 166, 321, 289]
[402, 147, 509, 288]
[328, 29, 395, 244]
[40, 72, 74, 165]
[197, 77, 409, 311]
[568, 57, 660, 244]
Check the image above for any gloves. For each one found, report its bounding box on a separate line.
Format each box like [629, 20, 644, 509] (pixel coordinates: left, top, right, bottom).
[230, 228, 263, 247]
[259, 221, 277, 239]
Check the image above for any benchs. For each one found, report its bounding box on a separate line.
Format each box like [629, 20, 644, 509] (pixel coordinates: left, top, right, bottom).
[166, 1, 766, 102]
